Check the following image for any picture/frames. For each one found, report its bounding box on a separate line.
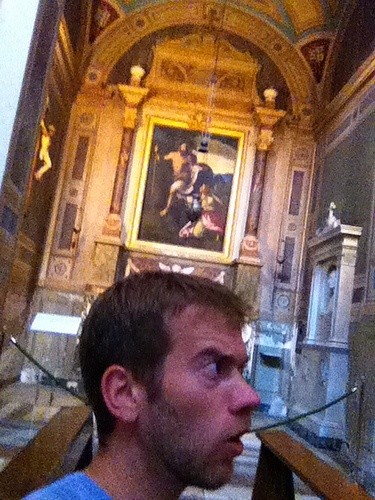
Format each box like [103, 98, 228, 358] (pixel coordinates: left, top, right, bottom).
[124, 110, 250, 266]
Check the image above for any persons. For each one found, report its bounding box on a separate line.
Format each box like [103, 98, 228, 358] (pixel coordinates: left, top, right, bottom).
[18, 269, 260, 499]
